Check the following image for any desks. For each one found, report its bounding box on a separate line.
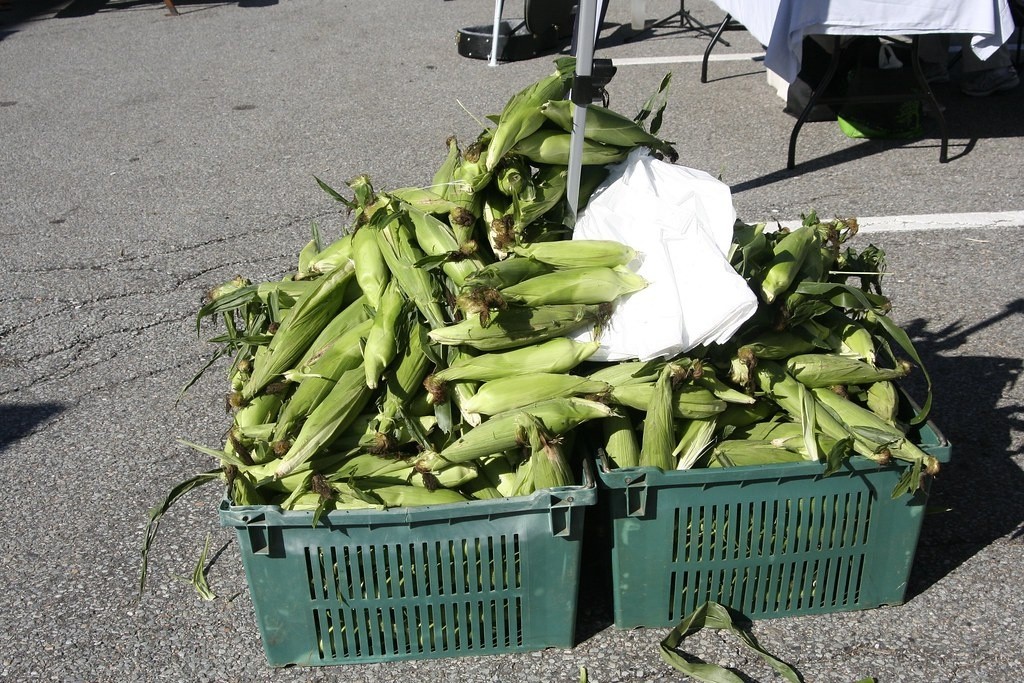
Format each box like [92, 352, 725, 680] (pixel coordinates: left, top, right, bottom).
[700, 0, 1018, 169]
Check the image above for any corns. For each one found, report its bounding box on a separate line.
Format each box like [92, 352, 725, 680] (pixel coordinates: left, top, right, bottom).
[193, 67, 940, 511]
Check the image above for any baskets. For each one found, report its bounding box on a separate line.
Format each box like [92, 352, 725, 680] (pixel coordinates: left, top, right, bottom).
[217, 473, 598, 658]
[578, 381, 952, 623]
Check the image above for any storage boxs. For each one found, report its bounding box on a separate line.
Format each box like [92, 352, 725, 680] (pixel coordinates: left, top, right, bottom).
[214, 430, 599, 670]
[594, 382, 954, 632]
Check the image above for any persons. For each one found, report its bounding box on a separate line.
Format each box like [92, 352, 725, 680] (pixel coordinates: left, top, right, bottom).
[916, 34, 1019, 97]
